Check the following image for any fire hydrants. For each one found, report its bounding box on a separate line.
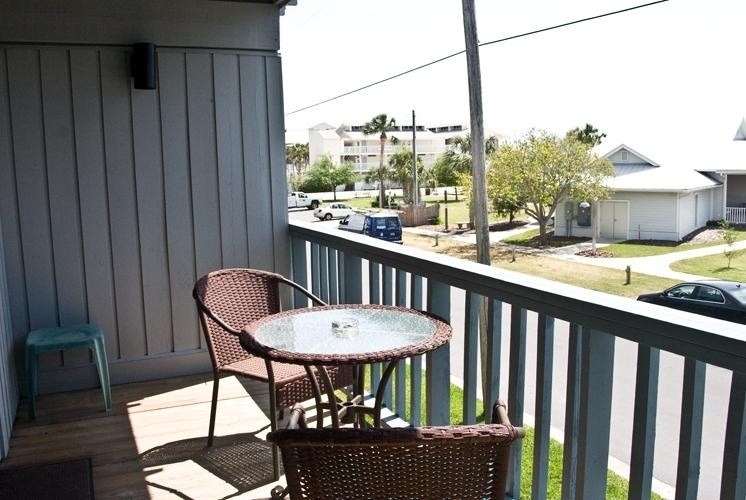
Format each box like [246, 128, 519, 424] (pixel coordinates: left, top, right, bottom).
[626, 266, 630, 284]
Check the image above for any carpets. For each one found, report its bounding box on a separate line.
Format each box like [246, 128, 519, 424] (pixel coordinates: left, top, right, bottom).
[0, 457, 93, 500]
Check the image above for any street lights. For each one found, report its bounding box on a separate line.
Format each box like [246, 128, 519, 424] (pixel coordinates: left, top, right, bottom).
[580, 199, 597, 255]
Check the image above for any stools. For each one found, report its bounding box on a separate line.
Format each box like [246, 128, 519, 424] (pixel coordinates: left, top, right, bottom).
[24, 324, 112, 420]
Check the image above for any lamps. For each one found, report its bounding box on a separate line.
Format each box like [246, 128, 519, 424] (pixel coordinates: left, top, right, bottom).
[128, 43, 156, 90]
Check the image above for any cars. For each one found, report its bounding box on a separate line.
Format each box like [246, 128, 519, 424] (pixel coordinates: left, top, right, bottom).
[636, 279, 745, 326]
[314, 202, 403, 244]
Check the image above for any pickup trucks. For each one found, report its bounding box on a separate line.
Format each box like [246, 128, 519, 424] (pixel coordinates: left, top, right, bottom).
[288, 192, 321, 209]
